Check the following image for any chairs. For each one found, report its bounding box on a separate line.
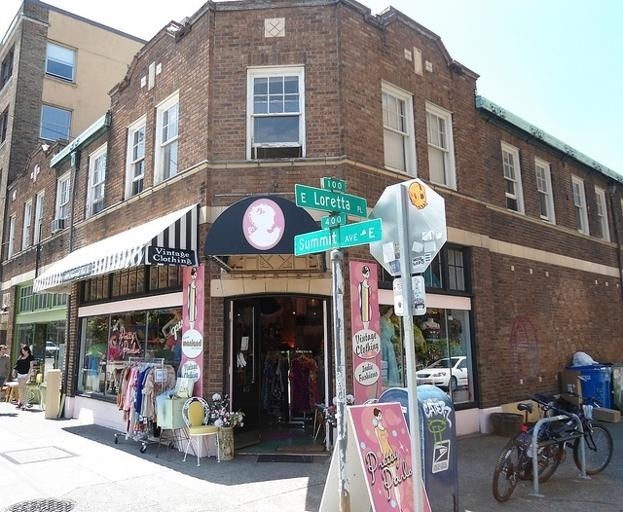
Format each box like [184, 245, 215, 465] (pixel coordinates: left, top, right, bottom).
[180, 396, 220, 467]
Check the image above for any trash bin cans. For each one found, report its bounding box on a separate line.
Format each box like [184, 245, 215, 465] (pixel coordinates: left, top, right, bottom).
[565, 363, 623, 416]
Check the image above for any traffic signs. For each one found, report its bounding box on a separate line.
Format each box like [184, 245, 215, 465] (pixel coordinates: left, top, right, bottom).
[293, 185, 369, 219]
[292, 218, 383, 258]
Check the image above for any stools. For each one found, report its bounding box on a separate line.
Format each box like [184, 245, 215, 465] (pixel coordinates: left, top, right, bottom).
[4, 381, 20, 403]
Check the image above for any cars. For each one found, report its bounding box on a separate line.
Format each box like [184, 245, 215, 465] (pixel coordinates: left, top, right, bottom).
[31, 342, 59, 356]
[416, 355, 469, 391]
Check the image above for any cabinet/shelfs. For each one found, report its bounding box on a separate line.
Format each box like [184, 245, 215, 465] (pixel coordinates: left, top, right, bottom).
[153, 395, 196, 462]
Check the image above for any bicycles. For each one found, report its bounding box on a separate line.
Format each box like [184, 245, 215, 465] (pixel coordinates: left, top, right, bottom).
[490, 391, 573, 503]
[522, 389, 613, 485]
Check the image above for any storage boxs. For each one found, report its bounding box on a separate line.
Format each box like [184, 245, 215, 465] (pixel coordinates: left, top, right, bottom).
[591, 405, 620, 423]
[558, 369, 583, 405]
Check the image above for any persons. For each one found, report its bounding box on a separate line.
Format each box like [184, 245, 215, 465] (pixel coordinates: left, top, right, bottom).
[0, 345, 10, 394]
[10, 344, 35, 409]
[161, 308, 181, 366]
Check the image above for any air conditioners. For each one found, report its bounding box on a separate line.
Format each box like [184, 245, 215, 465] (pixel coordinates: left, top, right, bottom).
[50, 219, 65, 234]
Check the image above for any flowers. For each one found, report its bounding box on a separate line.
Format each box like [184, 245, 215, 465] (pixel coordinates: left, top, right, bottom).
[206, 390, 246, 428]
[321, 393, 354, 428]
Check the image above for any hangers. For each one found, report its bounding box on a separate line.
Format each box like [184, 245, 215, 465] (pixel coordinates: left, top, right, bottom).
[267, 351, 313, 361]
[119, 358, 169, 373]
